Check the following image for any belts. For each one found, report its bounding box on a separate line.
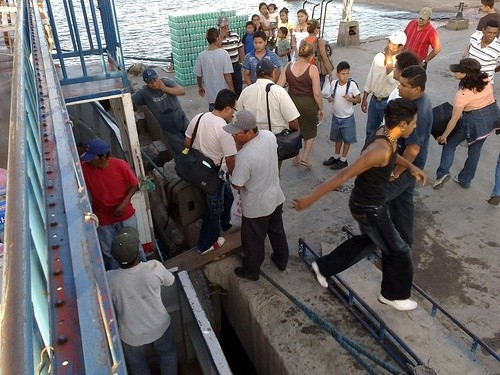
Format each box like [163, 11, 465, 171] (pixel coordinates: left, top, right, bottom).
[373, 94, 388, 101]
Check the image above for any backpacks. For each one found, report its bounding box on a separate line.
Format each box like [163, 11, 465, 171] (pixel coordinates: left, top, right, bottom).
[332, 78, 360, 105]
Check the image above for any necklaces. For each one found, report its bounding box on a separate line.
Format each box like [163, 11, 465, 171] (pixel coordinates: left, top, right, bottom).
[383, 127, 398, 142]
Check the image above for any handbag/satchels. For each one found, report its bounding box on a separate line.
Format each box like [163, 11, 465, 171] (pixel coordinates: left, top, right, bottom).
[173, 147, 226, 196]
[230, 193, 242, 229]
[315, 36, 335, 77]
[275, 129, 302, 161]
[431, 102, 463, 146]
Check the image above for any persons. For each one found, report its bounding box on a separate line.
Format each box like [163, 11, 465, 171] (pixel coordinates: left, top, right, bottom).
[103, 225, 181, 375]
[217, 17, 244, 100]
[487, 152, 500, 205]
[236, 59, 301, 178]
[288, 97, 427, 311]
[433, 58, 500, 190]
[194, 27, 237, 112]
[404, 7, 442, 81]
[226, 113, 291, 280]
[130, 68, 191, 155]
[380, 51, 425, 127]
[463, 20, 500, 87]
[476, 0, 500, 38]
[386, 65, 434, 250]
[322, 61, 362, 171]
[182, 88, 239, 255]
[360, 32, 407, 155]
[79, 136, 147, 270]
[240, 2, 334, 168]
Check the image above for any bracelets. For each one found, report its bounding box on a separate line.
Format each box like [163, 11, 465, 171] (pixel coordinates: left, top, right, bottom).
[422, 58, 428, 64]
[390, 171, 399, 179]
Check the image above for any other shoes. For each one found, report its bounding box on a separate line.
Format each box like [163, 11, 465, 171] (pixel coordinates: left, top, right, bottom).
[293, 157, 312, 168]
[453, 175, 470, 188]
[377, 292, 418, 312]
[323, 156, 348, 170]
[487, 196, 500, 205]
[234, 266, 259, 280]
[270, 252, 286, 271]
[432, 174, 451, 190]
[311, 261, 329, 288]
[198, 236, 226, 256]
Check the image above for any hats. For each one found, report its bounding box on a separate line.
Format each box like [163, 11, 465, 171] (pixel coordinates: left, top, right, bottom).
[110, 226, 139, 264]
[80, 138, 110, 162]
[256, 58, 273, 76]
[142, 68, 158, 81]
[450, 58, 482, 73]
[222, 110, 258, 134]
[218, 16, 230, 28]
[419, 7, 432, 21]
[386, 31, 407, 46]
